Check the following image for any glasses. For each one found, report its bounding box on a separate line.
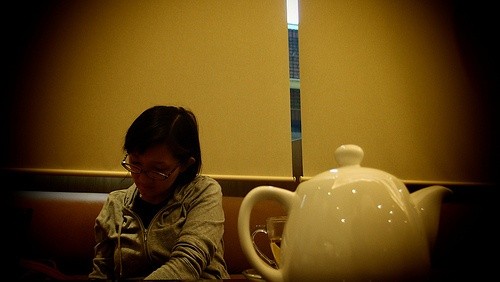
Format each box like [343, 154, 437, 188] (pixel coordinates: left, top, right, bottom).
[121, 153, 184, 180]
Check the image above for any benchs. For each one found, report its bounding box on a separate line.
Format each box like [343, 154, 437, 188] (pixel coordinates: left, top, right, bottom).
[0, 189, 290, 281]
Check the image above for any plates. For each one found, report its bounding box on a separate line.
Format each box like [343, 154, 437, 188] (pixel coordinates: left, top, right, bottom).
[242, 268, 267, 282]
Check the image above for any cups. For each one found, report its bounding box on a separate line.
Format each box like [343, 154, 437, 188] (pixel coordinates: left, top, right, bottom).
[249, 215, 288, 270]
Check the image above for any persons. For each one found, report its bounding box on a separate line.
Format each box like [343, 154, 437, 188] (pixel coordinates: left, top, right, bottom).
[87, 106, 230, 282]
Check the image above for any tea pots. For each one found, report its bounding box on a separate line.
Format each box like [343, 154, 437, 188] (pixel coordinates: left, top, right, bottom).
[237, 144, 452, 282]
